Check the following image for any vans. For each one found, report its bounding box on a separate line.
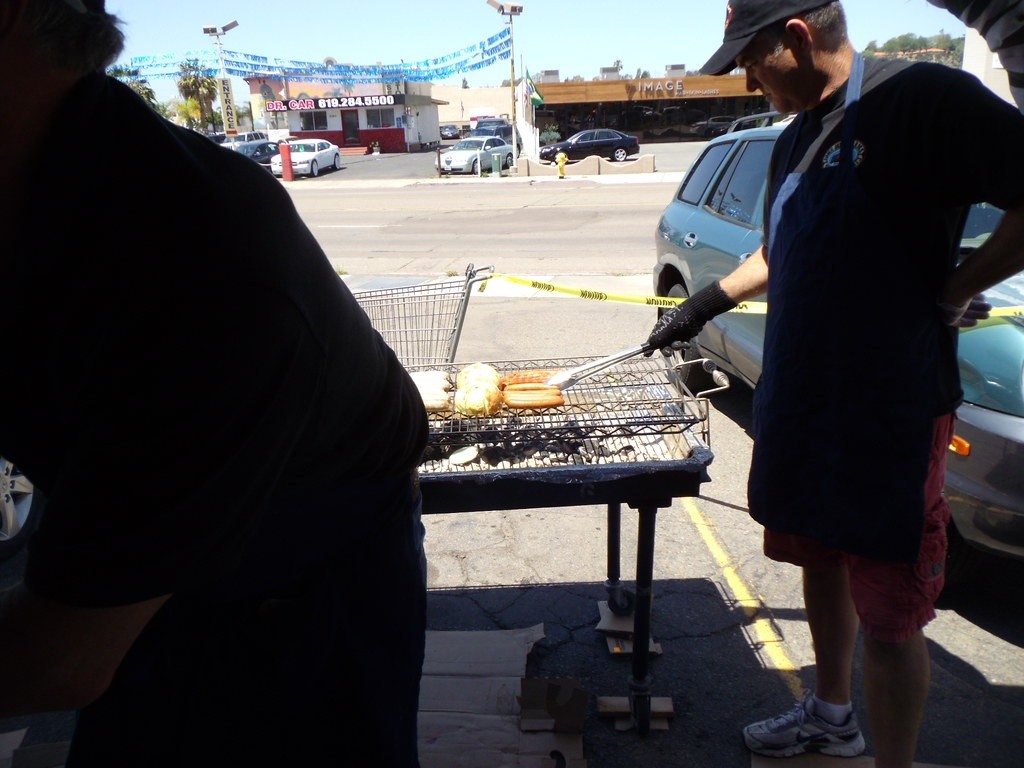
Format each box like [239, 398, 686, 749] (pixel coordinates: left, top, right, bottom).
[475, 118, 508, 128]
[204, 130, 269, 158]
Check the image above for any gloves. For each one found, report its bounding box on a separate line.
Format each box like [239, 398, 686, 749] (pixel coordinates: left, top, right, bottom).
[645, 281, 738, 357]
[947, 288, 993, 328]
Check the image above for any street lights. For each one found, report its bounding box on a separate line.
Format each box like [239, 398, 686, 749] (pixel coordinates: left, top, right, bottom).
[485, 0, 525, 172]
[202, 19, 238, 151]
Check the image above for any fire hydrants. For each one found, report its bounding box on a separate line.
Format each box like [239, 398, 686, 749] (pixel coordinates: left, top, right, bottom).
[556, 153, 569, 179]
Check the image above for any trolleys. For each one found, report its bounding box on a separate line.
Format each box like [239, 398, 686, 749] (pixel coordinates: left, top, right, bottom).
[345, 264, 497, 374]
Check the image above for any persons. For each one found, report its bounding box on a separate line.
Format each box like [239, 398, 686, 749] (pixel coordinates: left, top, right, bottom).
[0, 0, 430, 768]
[644, 1, 1023, 768]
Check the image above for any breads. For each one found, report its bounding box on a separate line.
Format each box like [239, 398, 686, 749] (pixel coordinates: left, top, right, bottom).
[454, 362, 504, 417]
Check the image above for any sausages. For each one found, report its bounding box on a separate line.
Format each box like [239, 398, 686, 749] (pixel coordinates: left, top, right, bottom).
[409, 370, 455, 412]
[500, 371, 565, 410]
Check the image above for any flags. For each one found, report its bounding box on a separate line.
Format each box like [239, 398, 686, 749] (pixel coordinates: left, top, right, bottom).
[526, 70, 544, 108]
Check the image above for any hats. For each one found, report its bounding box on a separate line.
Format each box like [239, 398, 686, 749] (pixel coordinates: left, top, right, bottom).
[697, 0, 837, 78]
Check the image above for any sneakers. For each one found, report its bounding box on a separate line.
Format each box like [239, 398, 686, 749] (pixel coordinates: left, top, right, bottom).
[744, 697, 865, 759]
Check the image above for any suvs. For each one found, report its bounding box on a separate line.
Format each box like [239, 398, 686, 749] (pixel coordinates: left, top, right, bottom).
[465, 123, 524, 159]
[649, 108, 1024, 609]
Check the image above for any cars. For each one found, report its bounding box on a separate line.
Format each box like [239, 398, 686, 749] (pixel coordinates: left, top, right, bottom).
[553, 102, 709, 142]
[440, 124, 460, 140]
[270, 138, 341, 178]
[235, 141, 279, 172]
[274, 135, 304, 152]
[539, 128, 641, 164]
[689, 115, 743, 140]
[434, 136, 513, 176]
